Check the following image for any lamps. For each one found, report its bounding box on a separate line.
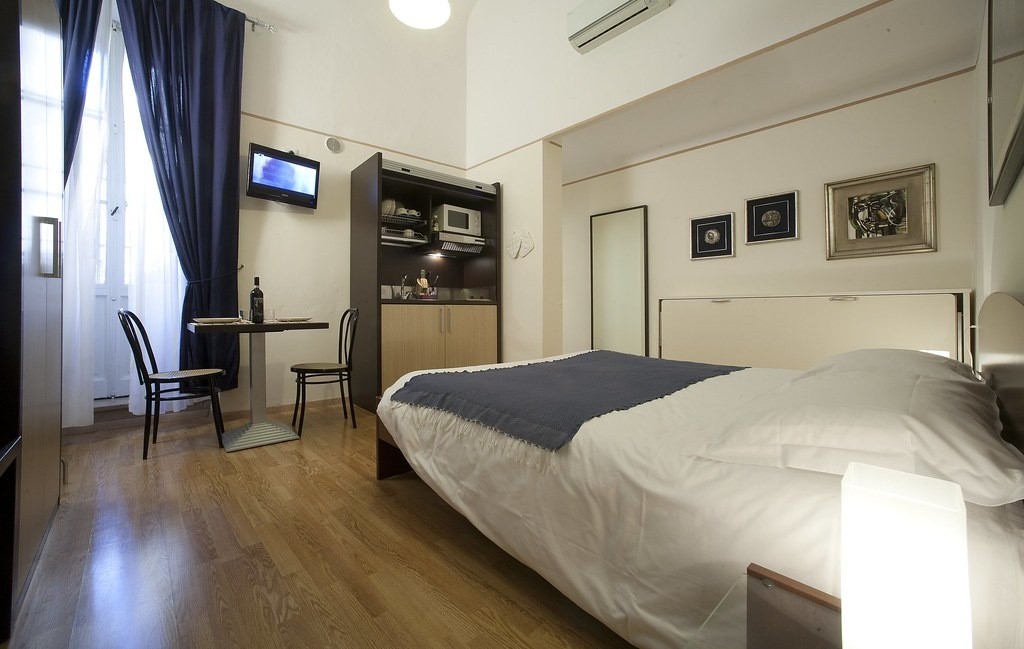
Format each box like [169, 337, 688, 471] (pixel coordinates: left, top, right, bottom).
[389, 0, 451, 31]
[840, 460, 973, 649]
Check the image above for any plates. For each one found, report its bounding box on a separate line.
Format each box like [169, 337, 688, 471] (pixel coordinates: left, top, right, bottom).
[193, 318, 240, 323]
[381, 199, 395, 215]
[275, 317, 312, 322]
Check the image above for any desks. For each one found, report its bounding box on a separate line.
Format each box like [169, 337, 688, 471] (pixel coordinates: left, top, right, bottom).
[185, 317, 330, 453]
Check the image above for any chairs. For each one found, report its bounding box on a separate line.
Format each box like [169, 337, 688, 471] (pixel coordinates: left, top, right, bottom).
[289, 307, 359, 440]
[118, 308, 227, 463]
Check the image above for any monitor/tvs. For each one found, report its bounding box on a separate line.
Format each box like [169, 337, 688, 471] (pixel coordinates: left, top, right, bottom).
[246, 143, 320, 209]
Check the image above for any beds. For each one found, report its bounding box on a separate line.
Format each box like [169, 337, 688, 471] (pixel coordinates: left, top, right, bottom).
[372, 288, 1024, 649]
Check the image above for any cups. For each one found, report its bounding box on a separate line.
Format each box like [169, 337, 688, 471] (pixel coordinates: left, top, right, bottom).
[264, 309, 275, 322]
[240, 309, 252, 323]
[409, 210, 421, 216]
[395, 208, 410, 216]
[403, 230, 414, 237]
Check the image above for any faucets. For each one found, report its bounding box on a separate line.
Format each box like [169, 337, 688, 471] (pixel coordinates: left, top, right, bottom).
[400, 272, 410, 299]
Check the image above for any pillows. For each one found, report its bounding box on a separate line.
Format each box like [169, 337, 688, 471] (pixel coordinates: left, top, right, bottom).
[695, 347, 1024, 509]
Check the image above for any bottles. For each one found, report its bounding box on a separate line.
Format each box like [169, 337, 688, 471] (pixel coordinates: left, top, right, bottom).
[250, 277, 264, 323]
[428, 272, 433, 293]
[433, 215, 439, 232]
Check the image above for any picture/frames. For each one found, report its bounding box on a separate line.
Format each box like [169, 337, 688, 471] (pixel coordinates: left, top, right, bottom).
[688, 210, 737, 262]
[824, 159, 939, 261]
[744, 189, 801, 245]
[986, 0, 1024, 208]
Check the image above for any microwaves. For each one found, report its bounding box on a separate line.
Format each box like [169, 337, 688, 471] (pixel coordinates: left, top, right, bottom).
[433, 204, 481, 237]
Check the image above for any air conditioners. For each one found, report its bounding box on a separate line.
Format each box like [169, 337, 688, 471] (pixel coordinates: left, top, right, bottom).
[564, 0, 672, 56]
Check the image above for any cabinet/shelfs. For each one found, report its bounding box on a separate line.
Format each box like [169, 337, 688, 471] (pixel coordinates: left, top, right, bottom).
[379, 304, 500, 389]
[5, 0, 66, 639]
[345, 151, 503, 302]
